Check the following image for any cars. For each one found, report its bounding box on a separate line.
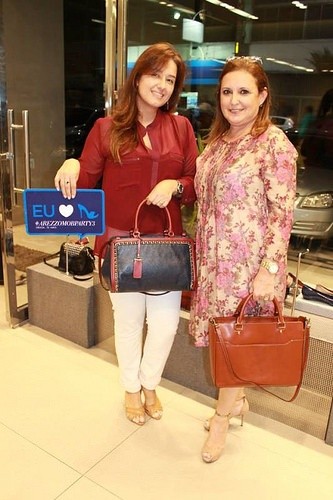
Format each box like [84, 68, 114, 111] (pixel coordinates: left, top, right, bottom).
[282, 125, 333, 247]
[65, 105, 105, 159]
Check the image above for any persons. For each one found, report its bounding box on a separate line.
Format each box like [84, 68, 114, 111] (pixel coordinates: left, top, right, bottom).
[271, 89, 333, 169]
[54, 42, 203, 426]
[174, 101, 214, 130]
[189, 55, 299, 462]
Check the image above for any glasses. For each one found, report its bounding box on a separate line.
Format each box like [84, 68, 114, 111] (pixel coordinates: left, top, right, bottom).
[226, 56, 264, 66]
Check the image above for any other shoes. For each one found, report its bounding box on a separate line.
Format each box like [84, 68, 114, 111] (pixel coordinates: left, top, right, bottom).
[301, 285, 333, 305]
[316, 283, 333, 295]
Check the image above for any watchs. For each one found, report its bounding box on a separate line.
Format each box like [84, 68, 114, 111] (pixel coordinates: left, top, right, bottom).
[261, 259, 278, 274]
[175, 180, 183, 197]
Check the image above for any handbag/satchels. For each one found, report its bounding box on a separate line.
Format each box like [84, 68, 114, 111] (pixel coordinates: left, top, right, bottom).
[207, 293, 313, 388]
[107, 199, 195, 293]
[57, 242, 95, 276]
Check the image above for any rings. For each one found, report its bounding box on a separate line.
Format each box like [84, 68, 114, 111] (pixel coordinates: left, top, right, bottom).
[264, 297, 269, 301]
[66, 181, 69, 184]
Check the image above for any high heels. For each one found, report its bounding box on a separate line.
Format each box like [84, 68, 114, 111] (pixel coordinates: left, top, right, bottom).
[140, 387, 164, 420]
[125, 390, 146, 426]
[203, 394, 249, 432]
[202, 411, 232, 464]
